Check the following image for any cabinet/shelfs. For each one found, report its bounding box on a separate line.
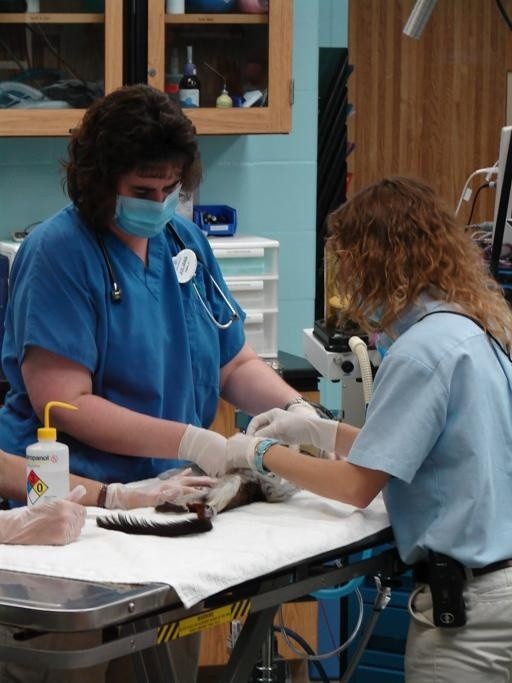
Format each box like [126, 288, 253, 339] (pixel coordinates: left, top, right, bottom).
[0, 234, 283, 381]
[0, 0, 295, 139]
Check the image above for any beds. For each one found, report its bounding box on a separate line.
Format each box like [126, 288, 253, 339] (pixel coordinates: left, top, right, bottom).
[0, 452, 406, 682]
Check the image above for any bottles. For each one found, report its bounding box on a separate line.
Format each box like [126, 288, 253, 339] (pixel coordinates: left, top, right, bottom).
[26, 400, 79, 508]
[164, 46, 200, 108]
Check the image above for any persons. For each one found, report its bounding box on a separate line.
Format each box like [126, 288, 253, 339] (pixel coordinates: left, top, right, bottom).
[2, 82, 322, 484]
[229, 173, 512, 683]
[2, 447, 216, 548]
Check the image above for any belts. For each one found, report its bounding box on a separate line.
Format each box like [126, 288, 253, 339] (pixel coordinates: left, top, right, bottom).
[413, 558, 512, 583]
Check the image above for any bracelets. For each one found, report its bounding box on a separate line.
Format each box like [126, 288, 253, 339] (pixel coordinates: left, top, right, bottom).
[283, 395, 309, 411]
[96, 483, 108, 509]
[254, 439, 284, 478]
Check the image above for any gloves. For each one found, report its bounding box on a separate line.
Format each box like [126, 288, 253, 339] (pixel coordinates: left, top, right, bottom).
[178, 400, 338, 479]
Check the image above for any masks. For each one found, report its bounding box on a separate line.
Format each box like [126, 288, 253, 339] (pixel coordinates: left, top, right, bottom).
[114, 178, 185, 236]
